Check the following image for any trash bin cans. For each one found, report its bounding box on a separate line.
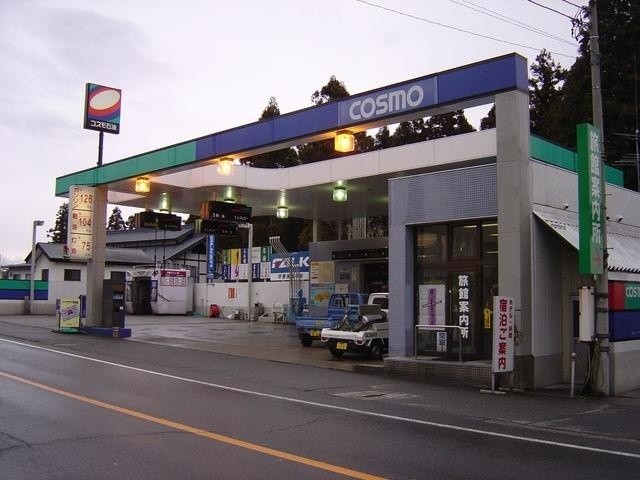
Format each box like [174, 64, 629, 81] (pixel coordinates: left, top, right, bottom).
[253, 302, 264, 321]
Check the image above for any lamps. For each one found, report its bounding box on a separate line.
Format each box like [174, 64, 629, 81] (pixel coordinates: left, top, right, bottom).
[218, 157, 234, 176]
[276, 206, 289, 219]
[134, 177, 150, 193]
[333, 129, 356, 152]
[332, 186, 348, 202]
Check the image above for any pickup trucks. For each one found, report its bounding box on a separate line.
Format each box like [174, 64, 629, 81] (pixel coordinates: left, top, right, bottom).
[295, 293, 389, 359]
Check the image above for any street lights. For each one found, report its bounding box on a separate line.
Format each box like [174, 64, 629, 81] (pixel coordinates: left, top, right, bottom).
[29, 221, 44, 315]
